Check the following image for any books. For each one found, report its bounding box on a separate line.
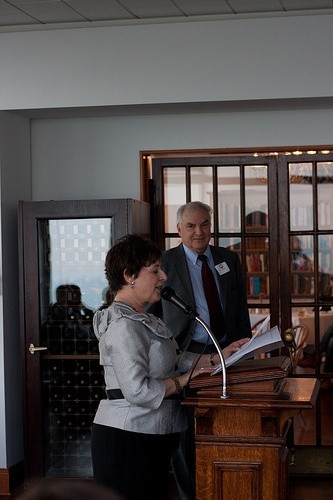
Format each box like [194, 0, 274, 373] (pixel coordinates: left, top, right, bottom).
[215, 201, 333, 312]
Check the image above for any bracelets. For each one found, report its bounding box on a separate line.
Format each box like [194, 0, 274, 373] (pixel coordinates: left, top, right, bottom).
[210, 353, 216, 366]
[170, 377, 181, 394]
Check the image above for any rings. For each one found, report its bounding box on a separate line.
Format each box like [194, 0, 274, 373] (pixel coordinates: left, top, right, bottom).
[200, 367, 207, 370]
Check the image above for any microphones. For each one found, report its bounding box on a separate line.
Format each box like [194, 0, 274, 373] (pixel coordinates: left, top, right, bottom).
[160, 286, 230, 400]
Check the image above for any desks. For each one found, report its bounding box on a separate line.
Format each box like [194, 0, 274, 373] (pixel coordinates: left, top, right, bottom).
[251, 307, 333, 359]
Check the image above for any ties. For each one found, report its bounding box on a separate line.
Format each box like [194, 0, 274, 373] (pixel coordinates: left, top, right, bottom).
[197, 255, 224, 342]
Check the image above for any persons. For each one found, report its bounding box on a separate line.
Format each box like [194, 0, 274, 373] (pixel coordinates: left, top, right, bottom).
[291, 235, 326, 303]
[146, 201, 254, 361]
[91, 233, 250, 500]
[224, 211, 269, 327]
[44, 285, 109, 382]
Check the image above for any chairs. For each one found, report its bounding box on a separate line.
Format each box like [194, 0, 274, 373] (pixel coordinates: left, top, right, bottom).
[252, 316, 333, 375]
